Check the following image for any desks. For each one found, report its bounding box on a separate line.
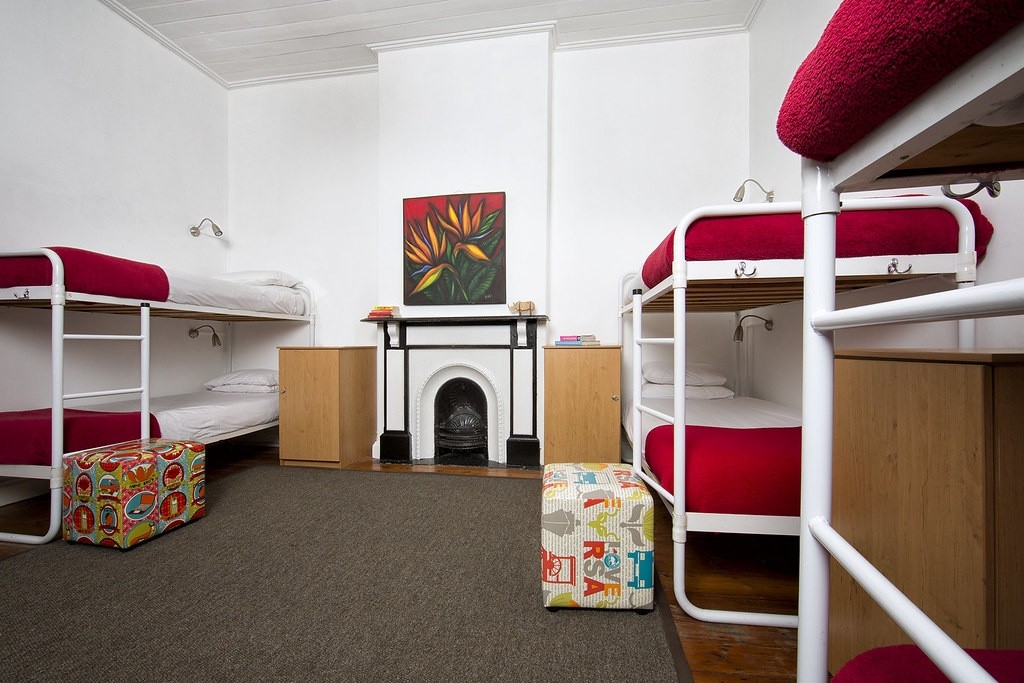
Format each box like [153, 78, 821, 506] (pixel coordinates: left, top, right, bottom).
[360, 316, 552, 465]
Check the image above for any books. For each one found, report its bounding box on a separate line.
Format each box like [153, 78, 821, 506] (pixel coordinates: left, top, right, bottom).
[367, 306, 401, 319]
[554, 335, 601, 346]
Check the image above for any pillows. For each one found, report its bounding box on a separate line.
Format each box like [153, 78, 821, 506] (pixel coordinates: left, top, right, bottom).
[212, 270, 300, 287]
[641, 362, 726, 386]
[204, 368, 278, 386]
[212, 385, 278, 393]
[733, 315, 773, 343]
[641, 383, 735, 400]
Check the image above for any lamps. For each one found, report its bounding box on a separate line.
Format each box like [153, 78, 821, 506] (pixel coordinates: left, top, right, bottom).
[733, 179, 774, 203]
[189, 324, 222, 347]
[190, 218, 223, 237]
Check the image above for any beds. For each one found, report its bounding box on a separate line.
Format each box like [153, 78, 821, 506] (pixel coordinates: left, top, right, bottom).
[618, 0, 1024, 683]
[0, 246, 317, 545]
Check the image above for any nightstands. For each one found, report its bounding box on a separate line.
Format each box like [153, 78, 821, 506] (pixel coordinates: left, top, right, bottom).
[543, 347, 624, 461]
[828, 347, 1024, 673]
[279, 345, 375, 472]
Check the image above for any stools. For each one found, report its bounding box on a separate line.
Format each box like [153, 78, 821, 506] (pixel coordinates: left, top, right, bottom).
[64, 438, 206, 549]
[541, 461, 654, 611]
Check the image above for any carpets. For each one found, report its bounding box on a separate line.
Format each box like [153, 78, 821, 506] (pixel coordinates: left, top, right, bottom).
[0, 464, 693, 683]
